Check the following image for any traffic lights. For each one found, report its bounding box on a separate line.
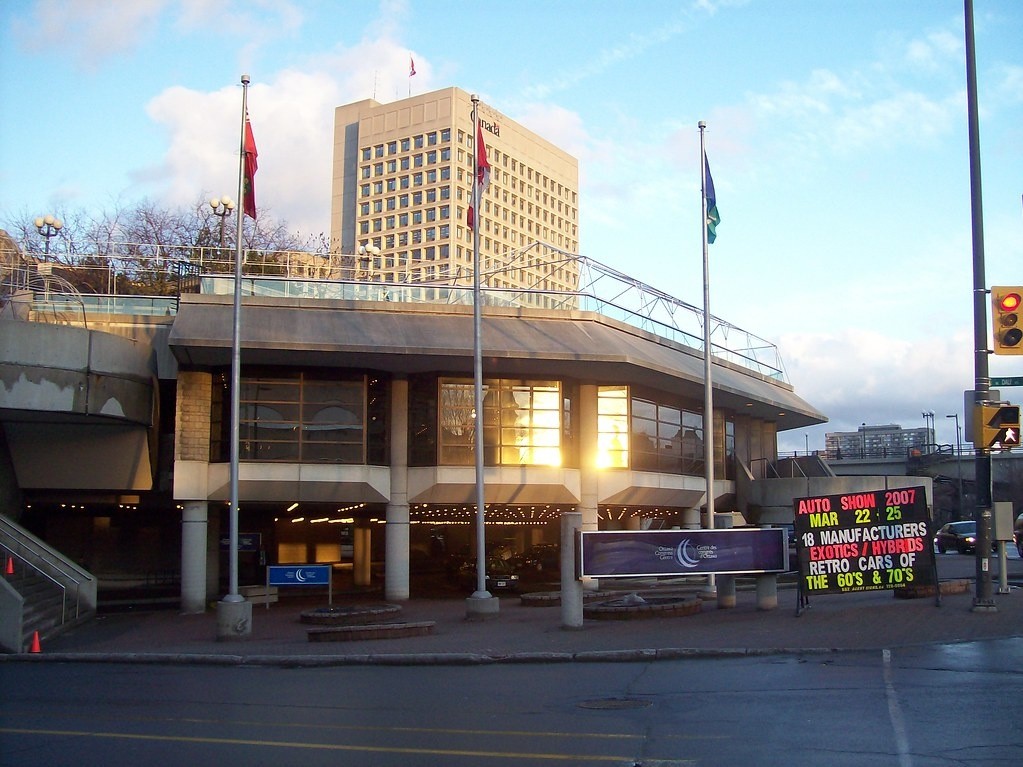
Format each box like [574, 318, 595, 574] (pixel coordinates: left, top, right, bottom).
[977, 407, 1002, 447]
[1000, 404, 1021, 447]
[991, 285, 1023, 356]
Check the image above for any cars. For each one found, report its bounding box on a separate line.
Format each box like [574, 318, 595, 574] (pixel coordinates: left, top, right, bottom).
[452, 556, 520, 595]
[515, 544, 559, 571]
[935, 521, 997, 554]
[340, 534, 354, 559]
[777, 524, 796, 543]
[1014, 513, 1022, 557]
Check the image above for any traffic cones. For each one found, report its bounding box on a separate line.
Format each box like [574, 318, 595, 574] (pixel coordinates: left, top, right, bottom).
[6, 557, 14, 576]
[28, 631, 43, 653]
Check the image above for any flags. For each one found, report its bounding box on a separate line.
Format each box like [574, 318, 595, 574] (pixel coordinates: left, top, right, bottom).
[409, 58, 416, 77]
[467, 116, 491, 232]
[244, 105, 257, 220]
[704, 149, 720, 244]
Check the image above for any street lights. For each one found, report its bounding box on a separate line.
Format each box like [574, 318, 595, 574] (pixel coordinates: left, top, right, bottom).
[959, 427, 962, 451]
[209, 196, 234, 262]
[947, 414, 964, 518]
[358, 244, 380, 298]
[862, 422, 867, 458]
[804, 432, 808, 456]
[35, 214, 62, 298]
[922, 409, 935, 454]
